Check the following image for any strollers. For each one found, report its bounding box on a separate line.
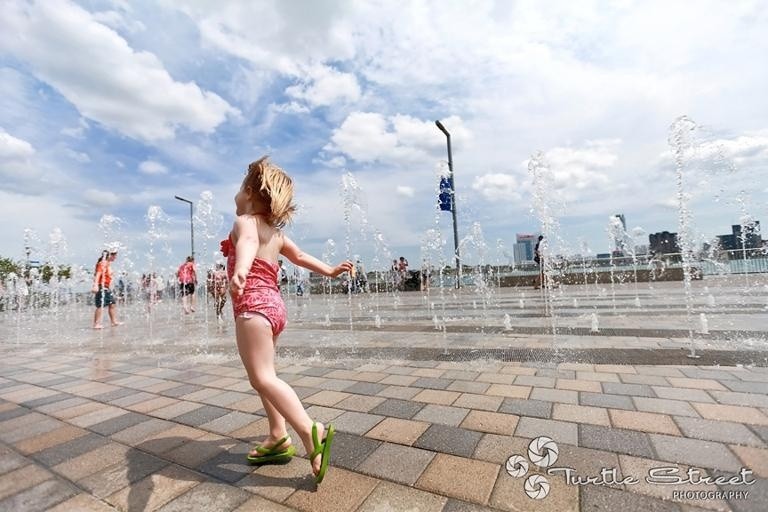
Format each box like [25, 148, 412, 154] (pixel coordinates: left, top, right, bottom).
[398, 270, 428, 291]
[342, 277, 366, 293]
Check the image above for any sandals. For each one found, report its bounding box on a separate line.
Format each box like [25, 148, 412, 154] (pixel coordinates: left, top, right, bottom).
[309, 422, 334, 483]
[247, 433, 295, 463]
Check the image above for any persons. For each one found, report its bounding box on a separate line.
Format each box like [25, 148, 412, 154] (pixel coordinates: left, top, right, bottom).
[92, 247, 433, 330]
[533, 235, 559, 290]
[218, 154, 352, 483]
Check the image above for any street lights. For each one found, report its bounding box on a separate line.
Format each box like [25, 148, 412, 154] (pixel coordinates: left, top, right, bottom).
[436, 119, 461, 287]
[177, 197, 194, 262]
